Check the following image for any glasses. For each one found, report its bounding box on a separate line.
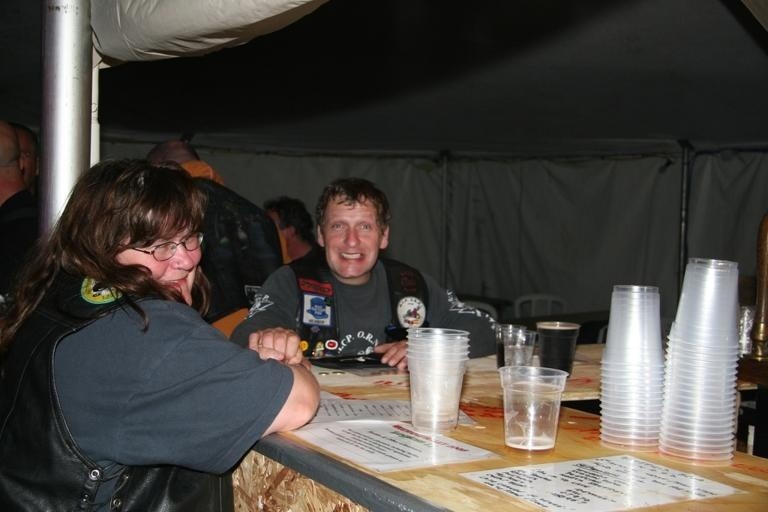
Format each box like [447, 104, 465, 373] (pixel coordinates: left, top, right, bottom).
[118, 232, 204, 262]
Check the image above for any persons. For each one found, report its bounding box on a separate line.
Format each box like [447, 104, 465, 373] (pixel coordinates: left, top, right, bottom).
[139, 140, 285, 345]
[0, 156, 322, 511]
[261, 198, 318, 267]
[228, 174, 510, 372]
[10, 123, 41, 204]
[0, 119, 40, 320]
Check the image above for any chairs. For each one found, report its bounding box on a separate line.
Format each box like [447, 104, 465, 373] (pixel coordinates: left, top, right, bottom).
[464, 300, 497, 322]
[514, 294, 567, 317]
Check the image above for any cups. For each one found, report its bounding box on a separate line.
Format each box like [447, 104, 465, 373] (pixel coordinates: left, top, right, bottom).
[495, 321, 581, 451]
[404, 327, 470, 433]
[599, 257, 739, 462]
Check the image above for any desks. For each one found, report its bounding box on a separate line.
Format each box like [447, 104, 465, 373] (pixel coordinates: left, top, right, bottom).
[497, 311, 613, 331]
